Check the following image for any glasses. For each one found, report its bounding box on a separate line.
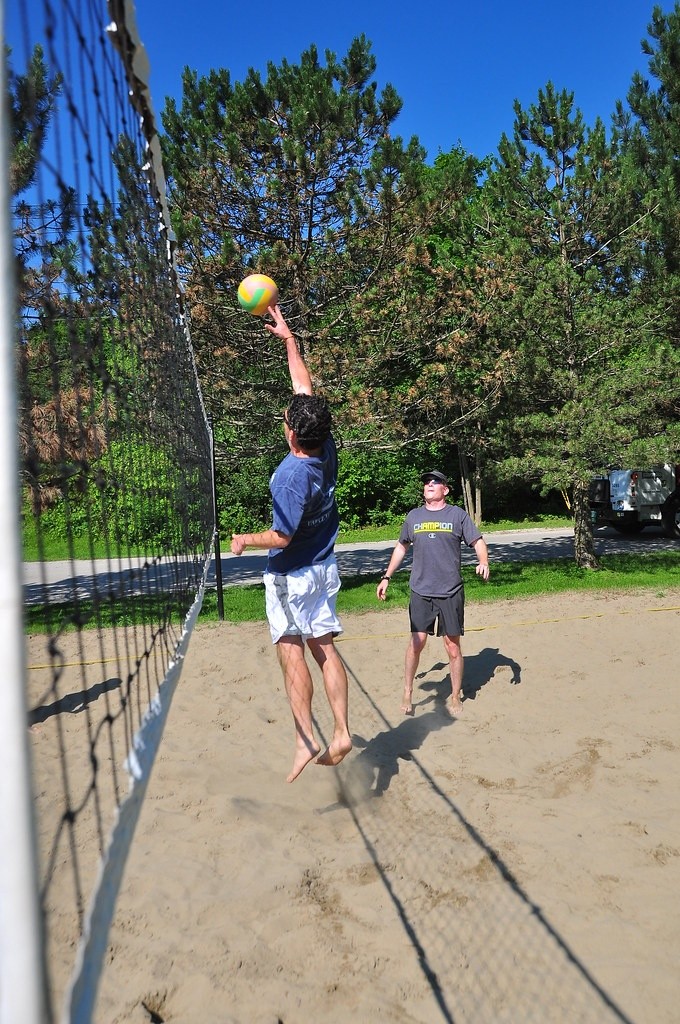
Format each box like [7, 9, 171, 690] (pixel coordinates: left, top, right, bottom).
[424, 478, 445, 486]
[281, 411, 290, 428]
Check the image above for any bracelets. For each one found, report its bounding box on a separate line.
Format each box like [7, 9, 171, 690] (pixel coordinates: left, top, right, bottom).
[381, 576, 390, 581]
[284, 335, 295, 341]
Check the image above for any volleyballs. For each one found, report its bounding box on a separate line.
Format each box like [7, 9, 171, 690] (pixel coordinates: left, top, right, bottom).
[237, 273, 279, 316]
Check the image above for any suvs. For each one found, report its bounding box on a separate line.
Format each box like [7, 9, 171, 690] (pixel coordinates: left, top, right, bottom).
[592, 462, 680, 539]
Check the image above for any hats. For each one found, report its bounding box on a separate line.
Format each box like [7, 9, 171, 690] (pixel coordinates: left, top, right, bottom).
[421, 471, 447, 485]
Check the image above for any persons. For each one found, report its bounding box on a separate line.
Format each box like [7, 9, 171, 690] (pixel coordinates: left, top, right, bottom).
[230, 306, 353, 784]
[376, 471, 489, 715]
